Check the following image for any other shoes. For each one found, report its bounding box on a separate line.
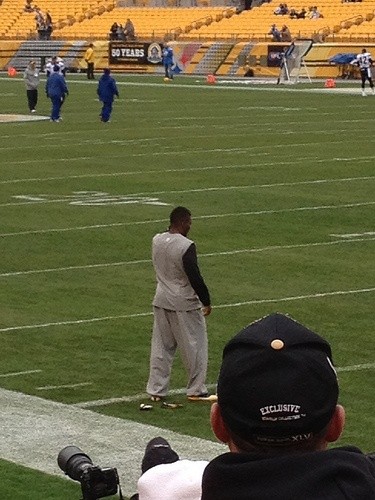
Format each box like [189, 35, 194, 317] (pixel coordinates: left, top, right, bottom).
[151, 395, 161, 401]
[189, 394, 217, 400]
[31, 108, 37, 112]
[362, 92, 367, 96]
[50, 116, 63, 122]
[372, 90, 375, 95]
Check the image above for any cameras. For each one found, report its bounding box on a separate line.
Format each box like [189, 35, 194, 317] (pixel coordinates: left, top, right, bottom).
[57, 445, 119, 500]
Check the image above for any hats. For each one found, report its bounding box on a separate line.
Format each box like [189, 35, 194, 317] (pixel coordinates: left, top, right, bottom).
[217, 312, 339, 448]
[141, 436, 179, 473]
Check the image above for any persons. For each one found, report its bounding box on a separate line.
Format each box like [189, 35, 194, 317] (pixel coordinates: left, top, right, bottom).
[116, 24, 127, 40]
[109, 22, 119, 40]
[23, 4, 55, 40]
[140, 437, 181, 475]
[24, 60, 40, 113]
[97, 67, 120, 124]
[84, 43, 98, 81]
[137, 311, 375, 499]
[272, 3, 322, 22]
[270, 24, 293, 44]
[350, 48, 375, 97]
[161, 43, 177, 82]
[45, 65, 69, 124]
[124, 18, 137, 39]
[145, 205, 220, 403]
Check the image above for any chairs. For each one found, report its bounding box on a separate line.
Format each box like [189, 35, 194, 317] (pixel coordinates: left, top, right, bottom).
[1, 0, 375, 77]
